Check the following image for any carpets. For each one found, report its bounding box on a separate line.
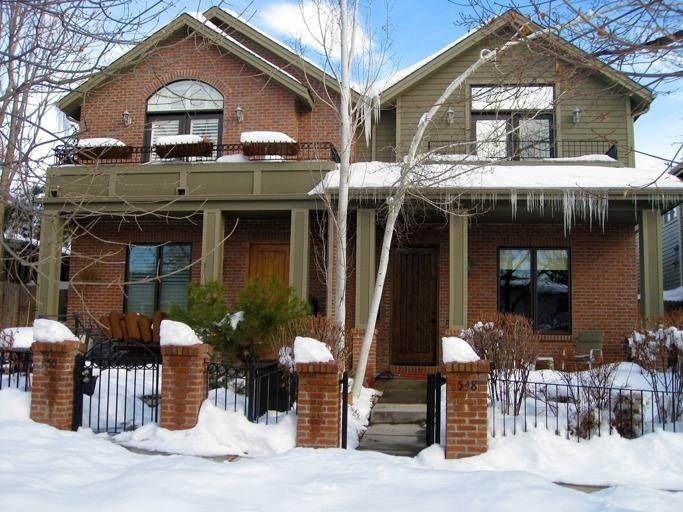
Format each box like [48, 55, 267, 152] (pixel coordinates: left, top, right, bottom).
[400, 373, 427, 379]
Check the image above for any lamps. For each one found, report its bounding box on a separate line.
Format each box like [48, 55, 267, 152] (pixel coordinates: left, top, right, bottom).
[446, 106, 455, 125]
[235, 104, 244, 123]
[123, 108, 132, 126]
[573, 106, 582, 123]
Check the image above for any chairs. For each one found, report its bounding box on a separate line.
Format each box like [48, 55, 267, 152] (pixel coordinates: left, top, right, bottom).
[97, 311, 164, 365]
[561, 330, 605, 372]
